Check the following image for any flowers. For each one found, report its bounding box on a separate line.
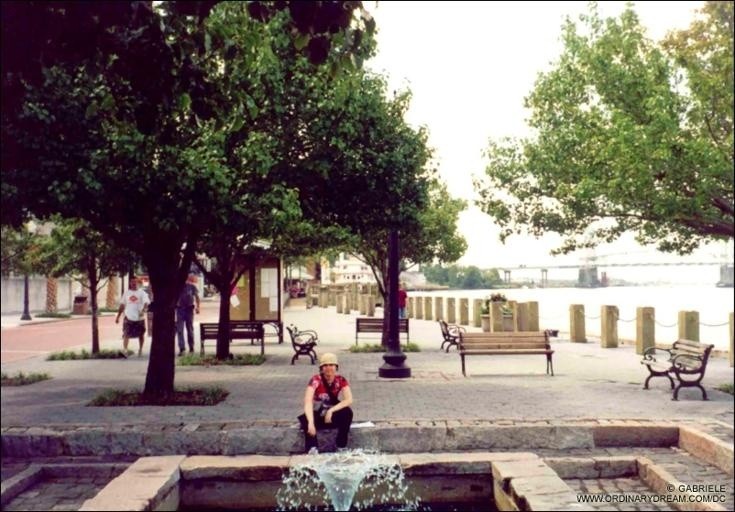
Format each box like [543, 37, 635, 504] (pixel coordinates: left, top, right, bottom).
[485, 293, 507, 309]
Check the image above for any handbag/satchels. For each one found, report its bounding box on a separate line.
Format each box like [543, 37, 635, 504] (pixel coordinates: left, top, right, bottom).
[333, 398, 340, 405]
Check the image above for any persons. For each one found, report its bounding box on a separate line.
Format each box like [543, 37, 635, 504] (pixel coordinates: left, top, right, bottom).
[297, 353, 353, 455]
[115, 276, 150, 358]
[398, 283, 407, 319]
[175, 283, 200, 356]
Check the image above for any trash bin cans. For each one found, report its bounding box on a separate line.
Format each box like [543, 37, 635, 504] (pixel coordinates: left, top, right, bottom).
[73, 296, 88, 315]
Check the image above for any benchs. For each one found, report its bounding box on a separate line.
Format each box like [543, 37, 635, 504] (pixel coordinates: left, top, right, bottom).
[459, 332, 555, 376]
[437, 319, 465, 353]
[355, 317, 409, 345]
[640, 338, 714, 402]
[200, 319, 318, 365]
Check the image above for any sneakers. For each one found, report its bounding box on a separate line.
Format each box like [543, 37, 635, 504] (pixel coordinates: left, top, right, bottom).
[307, 447, 318, 455]
[178, 349, 194, 357]
[336, 447, 348, 453]
[119, 352, 128, 359]
[138, 350, 143, 357]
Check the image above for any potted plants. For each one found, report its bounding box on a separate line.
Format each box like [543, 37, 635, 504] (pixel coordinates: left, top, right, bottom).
[479, 304, 490, 330]
[502, 306, 514, 331]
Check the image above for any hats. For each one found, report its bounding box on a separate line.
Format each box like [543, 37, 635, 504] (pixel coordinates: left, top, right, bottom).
[319, 353, 338, 367]
